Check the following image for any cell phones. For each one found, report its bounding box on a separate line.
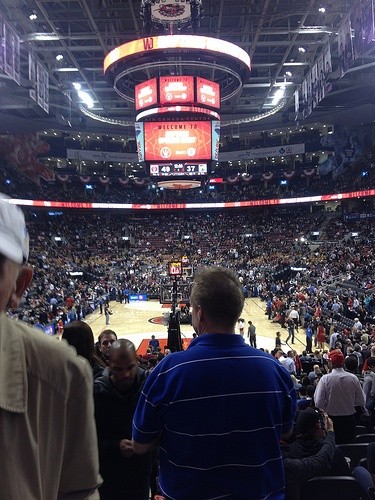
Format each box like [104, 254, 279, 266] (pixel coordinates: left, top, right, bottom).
[323, 413, 329, 431]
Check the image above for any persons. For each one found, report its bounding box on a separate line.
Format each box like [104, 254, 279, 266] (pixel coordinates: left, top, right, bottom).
[0, 141, 375, 500]
[0, 195, 103, 500]
[130, 265, 298, 500]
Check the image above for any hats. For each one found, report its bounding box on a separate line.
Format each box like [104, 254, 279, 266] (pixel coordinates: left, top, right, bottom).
[295, 408, 322, 435]
[322, 349, 346, 365]
[0, 192, 31, 264]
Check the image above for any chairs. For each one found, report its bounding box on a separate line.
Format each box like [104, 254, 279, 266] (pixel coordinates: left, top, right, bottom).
[298, 422, 375, 500]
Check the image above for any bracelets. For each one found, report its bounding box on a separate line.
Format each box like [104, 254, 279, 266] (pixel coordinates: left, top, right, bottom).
[327, 428, 334, 433]
